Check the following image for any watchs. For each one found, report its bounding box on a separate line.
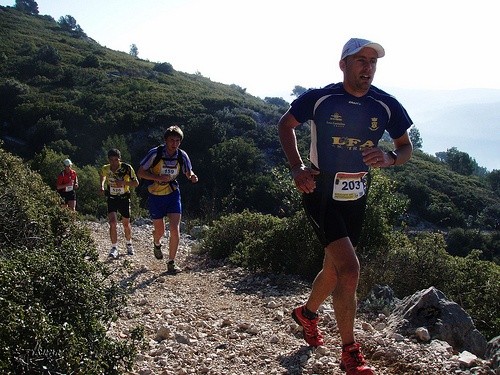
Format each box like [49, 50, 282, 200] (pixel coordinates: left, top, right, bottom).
[388, 151, 397, 165]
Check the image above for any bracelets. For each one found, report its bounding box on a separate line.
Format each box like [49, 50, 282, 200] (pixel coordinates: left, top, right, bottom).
[290, 162, 303, 172]
[101, 186, 104, 187]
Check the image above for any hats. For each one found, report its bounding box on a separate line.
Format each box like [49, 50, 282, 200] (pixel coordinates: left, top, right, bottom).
[341, 38, 385, 59]
[63, 159, 73, 166]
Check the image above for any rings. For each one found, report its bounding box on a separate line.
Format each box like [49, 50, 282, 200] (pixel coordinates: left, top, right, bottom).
[376, 156, 379, 162]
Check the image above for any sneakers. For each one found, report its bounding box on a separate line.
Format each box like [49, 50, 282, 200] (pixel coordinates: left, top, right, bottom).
[168, 260, 182, 273]
[340, 344, 376, 375]
[126, 244, 134, 255]
[292, 305, 324, 346]
[108, 249, 118, 258]
[154, 242, 163, 259]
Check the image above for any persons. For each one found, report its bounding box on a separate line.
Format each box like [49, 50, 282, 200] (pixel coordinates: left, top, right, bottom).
[99, 149, 140, 259]
[278, 38, 413, 375]
[138, 125, 198, 273]
[55, 159, 79, 222]
